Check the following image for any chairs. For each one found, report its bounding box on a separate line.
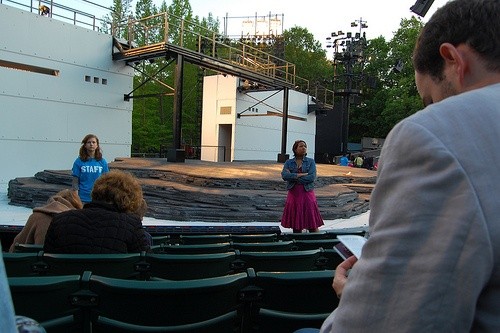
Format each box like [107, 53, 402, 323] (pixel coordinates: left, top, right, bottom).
[0, 231, 367, 333]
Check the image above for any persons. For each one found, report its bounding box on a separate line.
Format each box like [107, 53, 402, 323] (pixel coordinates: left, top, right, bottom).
[44, 170, 152, 255]
[320, 0, 500, 333]
[281, 140, 324, 233]
[72, 134, 109, 206]
[339, 153, 374, 170]
[9, 189, 83, 253]
